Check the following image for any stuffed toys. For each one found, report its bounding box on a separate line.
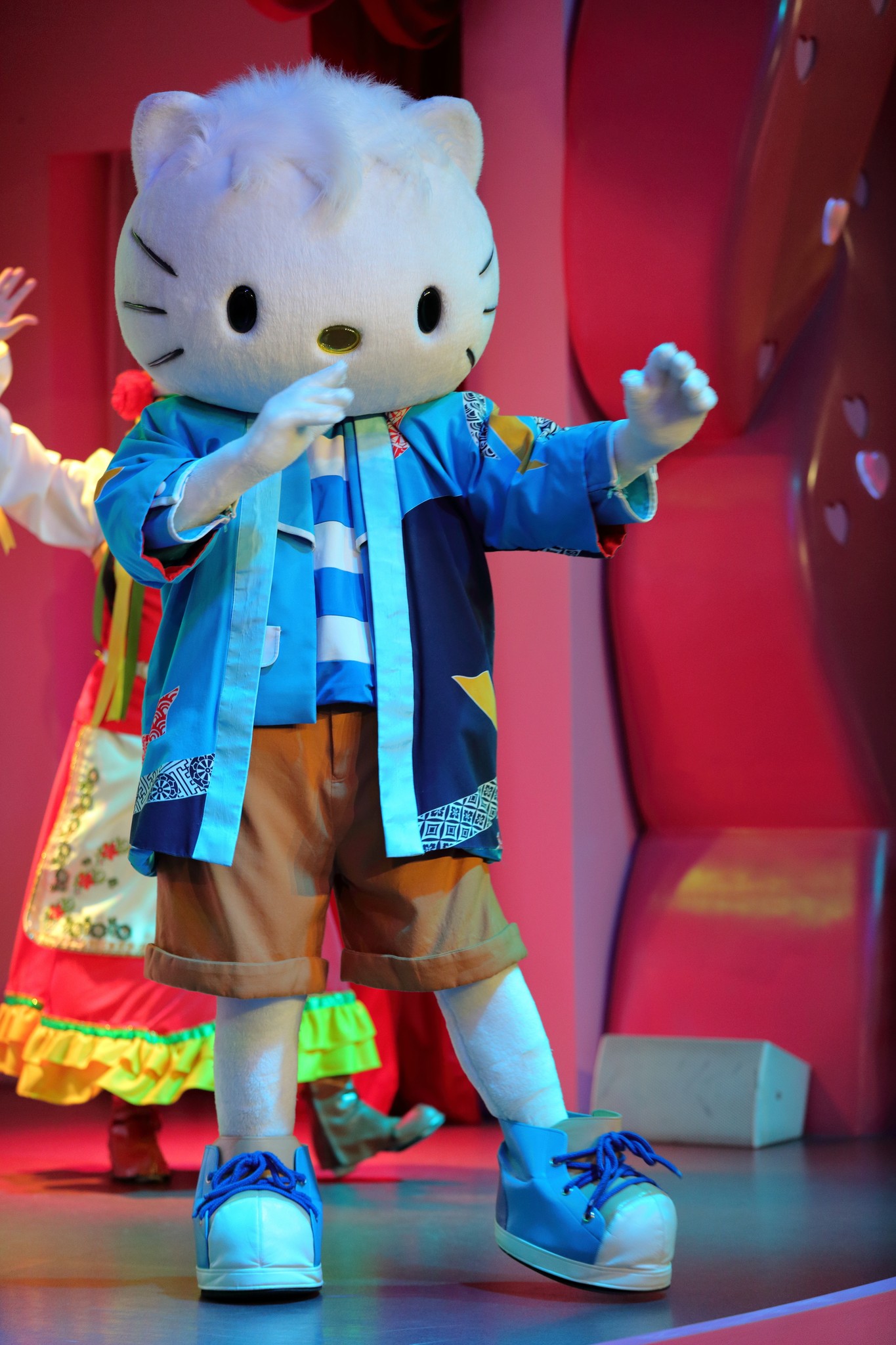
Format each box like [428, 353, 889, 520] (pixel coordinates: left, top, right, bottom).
[95, 56, 719, 1295]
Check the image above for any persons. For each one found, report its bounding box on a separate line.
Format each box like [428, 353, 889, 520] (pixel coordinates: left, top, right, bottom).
[1, 266, 120, 559]
[0, 371, 447, 1186]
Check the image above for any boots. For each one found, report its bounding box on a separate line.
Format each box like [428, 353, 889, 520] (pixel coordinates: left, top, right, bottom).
[107, 1094, 173, 1185]
[301, 1074, 445, 1178]
